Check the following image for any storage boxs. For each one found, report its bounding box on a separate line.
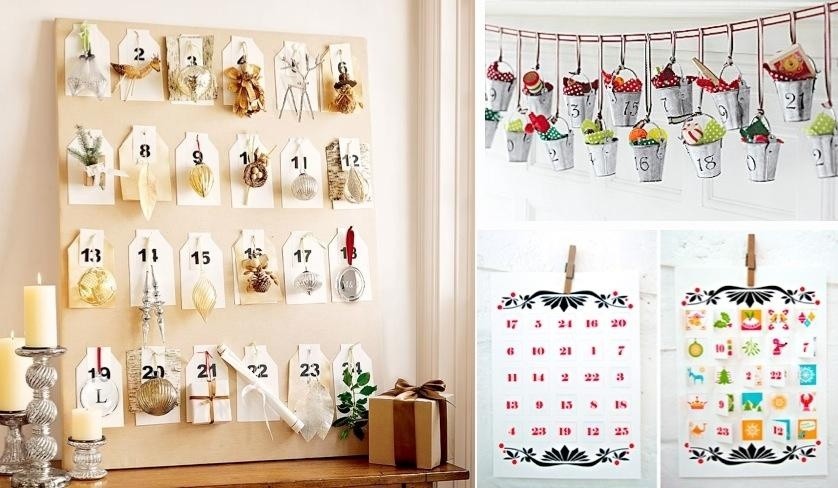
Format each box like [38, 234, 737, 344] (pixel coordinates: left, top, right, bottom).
[368, 391, 452, 472]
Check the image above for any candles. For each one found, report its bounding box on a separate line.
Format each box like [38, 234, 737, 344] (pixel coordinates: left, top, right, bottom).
[2, 336, 37, 412]
[71, 406, 101, 440]
[20, 285, 55, 346]
